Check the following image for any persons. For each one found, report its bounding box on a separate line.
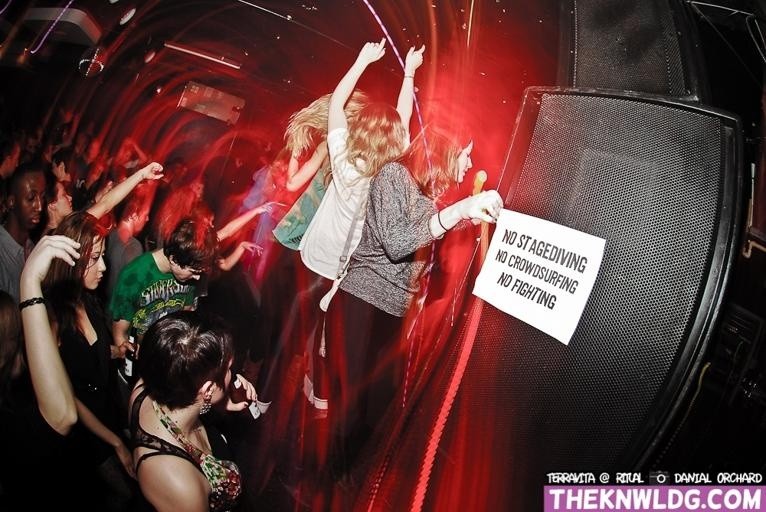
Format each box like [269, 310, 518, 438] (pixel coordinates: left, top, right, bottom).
[0, 38, 505, 512]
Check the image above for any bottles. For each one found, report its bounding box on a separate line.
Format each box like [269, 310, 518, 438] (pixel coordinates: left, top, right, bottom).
[227, 371, 264, 426]
[125, 327, 139, 380]
